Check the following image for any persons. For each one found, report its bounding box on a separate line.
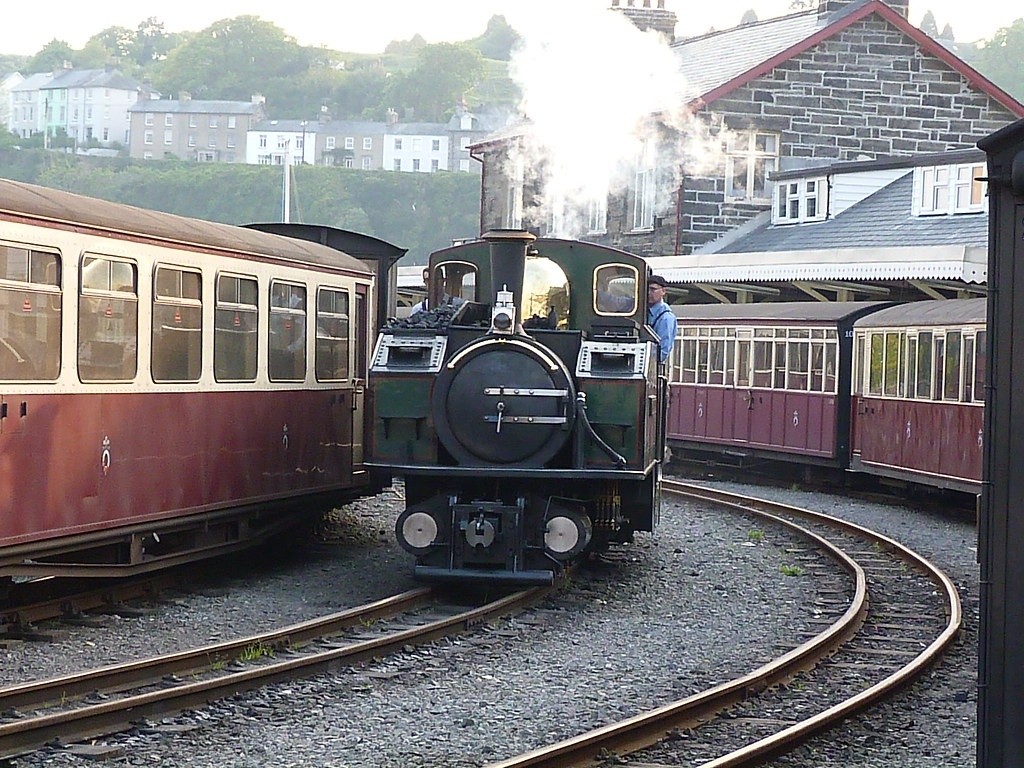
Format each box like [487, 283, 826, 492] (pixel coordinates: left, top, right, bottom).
[247, 284, 310, 379]
[595, 274, 678, 362]
[409, 267, 466, 317]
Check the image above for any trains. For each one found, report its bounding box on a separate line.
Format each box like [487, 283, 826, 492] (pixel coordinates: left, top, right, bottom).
[664, 296, 987, 508]
[0, 178, 412, 604]
[349, 129, 675, 599]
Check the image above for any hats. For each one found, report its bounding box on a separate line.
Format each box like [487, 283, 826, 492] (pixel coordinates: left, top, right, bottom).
[647, 275, 667, 287]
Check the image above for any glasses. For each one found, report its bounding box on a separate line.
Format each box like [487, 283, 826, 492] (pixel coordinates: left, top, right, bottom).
[648, 286, 663, 292]
